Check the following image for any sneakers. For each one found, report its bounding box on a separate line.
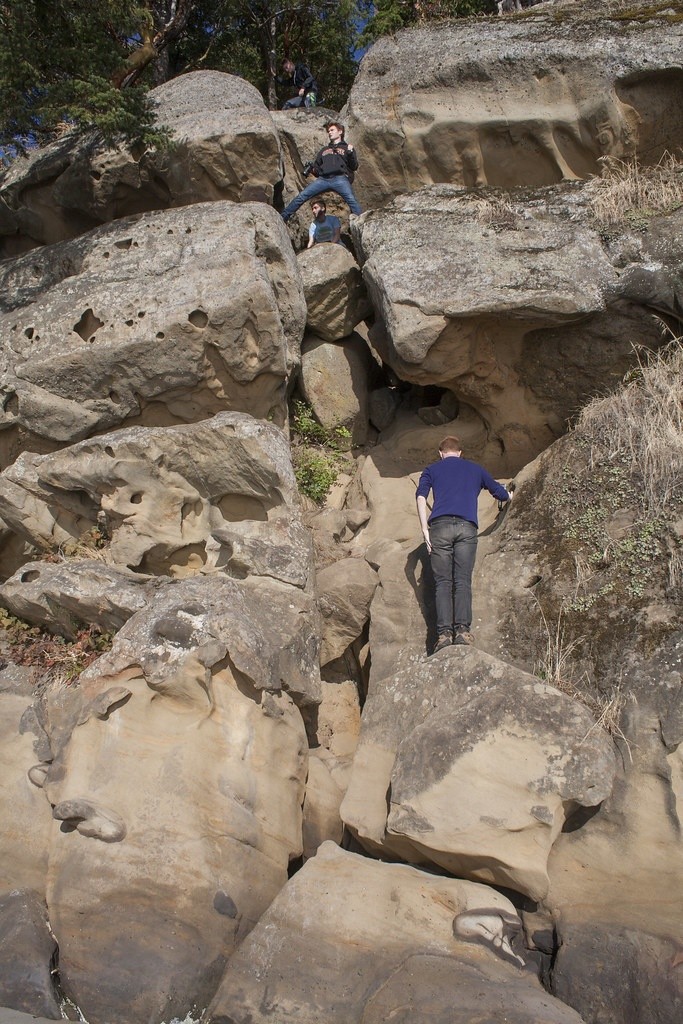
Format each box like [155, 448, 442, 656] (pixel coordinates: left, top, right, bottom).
[433, 631, 453, 652]
[455, 626, 474, 644]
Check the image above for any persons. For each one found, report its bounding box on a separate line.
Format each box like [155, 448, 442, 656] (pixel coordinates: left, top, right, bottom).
[415, 436, 513, 652]
[282, 123, 362, 223]
[279, 59, 317, 108]
[306, 199, 346, 247]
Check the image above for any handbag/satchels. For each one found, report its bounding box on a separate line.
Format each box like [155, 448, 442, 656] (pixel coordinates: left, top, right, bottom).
[349, 171, 354, 185]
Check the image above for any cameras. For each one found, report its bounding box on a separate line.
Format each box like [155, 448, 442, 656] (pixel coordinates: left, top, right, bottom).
[301, 161, 314, 176]
